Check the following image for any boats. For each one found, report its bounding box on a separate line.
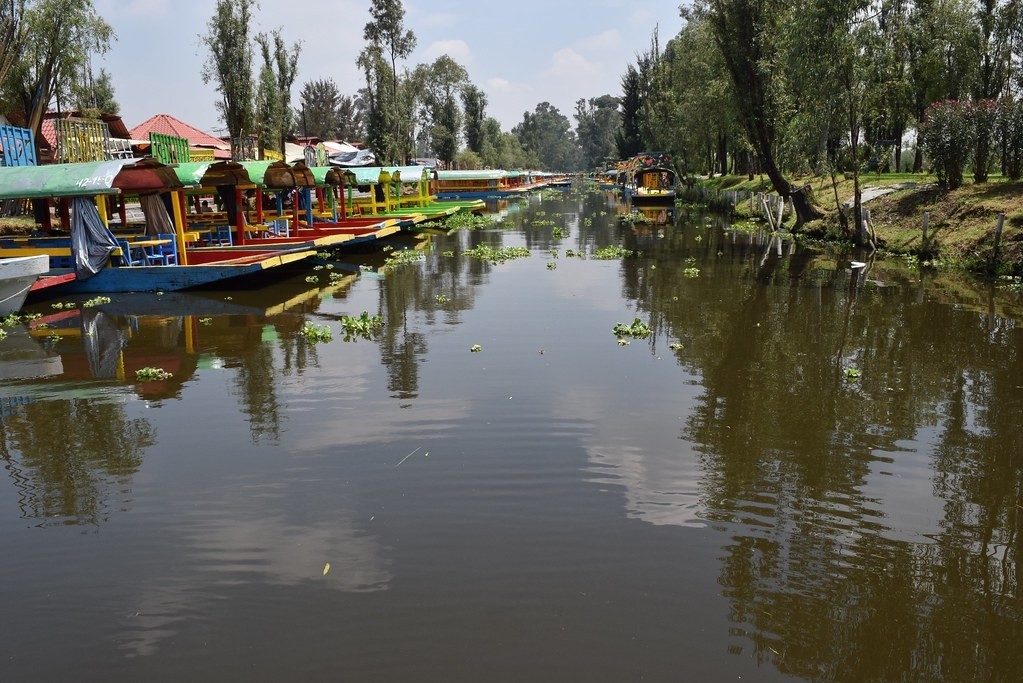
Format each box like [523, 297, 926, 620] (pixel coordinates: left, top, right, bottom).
[25, 272, 76, 303]
[23, 233, 436, 405]
[0, 156, 571, 291]
[581, 154, 677, 209]
[0, 254, 51, 319]
[616, 202, 675, 242]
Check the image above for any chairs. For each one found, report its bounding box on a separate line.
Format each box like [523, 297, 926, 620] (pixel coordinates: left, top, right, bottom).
[110, 223, 147, 234]
[263, 220, 289, 239]
[218, 226, 232, 247]
[134, 236, 154, 266]
[197, 232, 212, 248]
[129, 240, 172, 267]
[118, 241, 144, 267]
[1, 229, 70, 248]
[158, 234, 178, 267]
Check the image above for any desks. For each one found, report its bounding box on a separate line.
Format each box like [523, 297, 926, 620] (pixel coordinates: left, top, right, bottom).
[187, 211, 263, 223]
[263, 208, 294, 218]
[298, 208, 355, 217]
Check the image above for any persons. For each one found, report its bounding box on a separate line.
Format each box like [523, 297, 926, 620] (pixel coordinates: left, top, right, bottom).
[200, 200, 213, 212]
